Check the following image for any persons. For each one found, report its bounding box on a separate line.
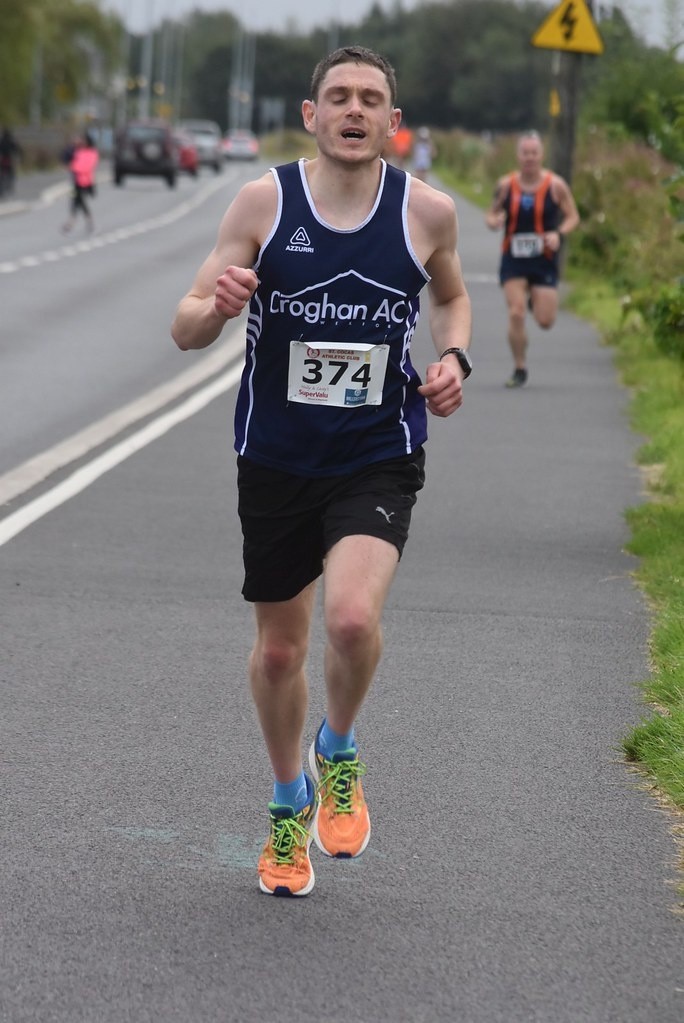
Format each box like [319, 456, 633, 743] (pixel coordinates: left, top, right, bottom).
[170, 46, 473, 897]
[392, 120, 438, 182]
[61, 129, 98, 238]
[487, 135, 580, 387]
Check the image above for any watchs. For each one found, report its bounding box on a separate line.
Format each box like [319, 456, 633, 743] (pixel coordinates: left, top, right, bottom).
[440, 348, 473, 380]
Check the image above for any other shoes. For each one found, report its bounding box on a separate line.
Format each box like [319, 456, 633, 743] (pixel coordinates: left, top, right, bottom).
[507, 371, 526, 387]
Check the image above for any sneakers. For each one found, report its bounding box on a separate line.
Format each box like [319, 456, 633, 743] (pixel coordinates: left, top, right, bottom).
[258, 772, 321, 896]
[307, 718, 372, 858]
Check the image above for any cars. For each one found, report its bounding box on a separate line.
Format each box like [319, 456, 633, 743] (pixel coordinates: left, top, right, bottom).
[174, 131, 199, 177]
[223, 126, 261, 162]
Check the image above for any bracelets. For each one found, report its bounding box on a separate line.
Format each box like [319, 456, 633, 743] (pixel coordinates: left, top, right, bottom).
[554, 228, 564, 245]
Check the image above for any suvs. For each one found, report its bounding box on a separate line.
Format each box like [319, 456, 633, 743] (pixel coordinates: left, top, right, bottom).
[112, 115, 180, 189]
[170, 117, 225, 173]
